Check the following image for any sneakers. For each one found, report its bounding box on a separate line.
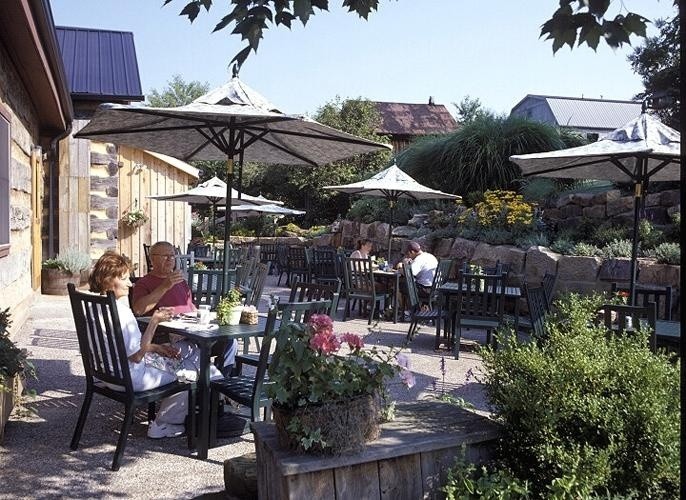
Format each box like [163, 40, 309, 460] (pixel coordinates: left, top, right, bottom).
[147, 419, 186, 439]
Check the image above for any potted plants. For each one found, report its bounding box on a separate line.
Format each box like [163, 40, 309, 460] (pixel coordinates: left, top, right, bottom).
[42, 258, 80, 295]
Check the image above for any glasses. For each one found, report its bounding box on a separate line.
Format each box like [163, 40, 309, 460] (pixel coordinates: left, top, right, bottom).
[151, 253, 176, 259]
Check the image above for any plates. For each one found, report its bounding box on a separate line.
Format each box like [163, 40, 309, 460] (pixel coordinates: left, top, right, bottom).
[177, 312, 197, 321]
[185, 324, 219, 331]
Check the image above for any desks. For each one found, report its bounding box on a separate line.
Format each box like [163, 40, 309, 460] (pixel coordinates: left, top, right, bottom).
[612, 315, 681, 355]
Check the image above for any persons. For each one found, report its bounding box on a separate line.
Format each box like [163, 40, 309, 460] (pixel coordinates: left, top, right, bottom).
[82, 250, 215, 439]
[395, 241, 443, 321]
[349, 239, 385, 310]
[129, 241, 239, 415]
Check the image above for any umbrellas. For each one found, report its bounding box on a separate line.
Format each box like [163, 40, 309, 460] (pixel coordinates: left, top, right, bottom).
[323, 156, 463, 259]
[71, 60, 393, 413]
[507, 100, 681, 305]
[145, 171, 285, 267]
[217, 190, 307, 244]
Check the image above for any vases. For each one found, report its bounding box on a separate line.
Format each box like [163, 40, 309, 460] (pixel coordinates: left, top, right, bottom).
[126, 222, 143, 229]
[486, 235, 515, 245]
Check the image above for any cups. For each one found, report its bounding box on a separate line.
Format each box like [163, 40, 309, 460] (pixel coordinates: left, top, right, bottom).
[379, 264, 393, 272]
[198, 305, 211, 312]
[196, 310, 210, 326]
[159, 306, 175, 319]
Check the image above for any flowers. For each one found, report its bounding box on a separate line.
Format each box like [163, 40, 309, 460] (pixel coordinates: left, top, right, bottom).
[458, 189, 535, 238]
[119, 209, 149, 226]
